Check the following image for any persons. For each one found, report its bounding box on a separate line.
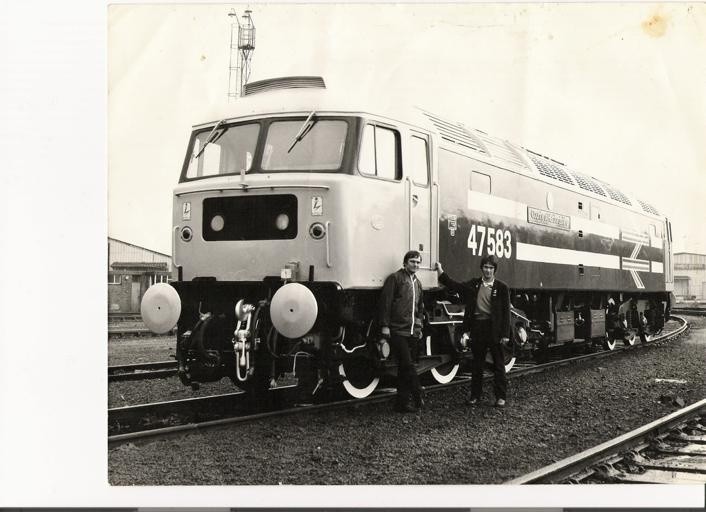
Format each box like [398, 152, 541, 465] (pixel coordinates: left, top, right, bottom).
[434, 254, 511, 408]
[381, 250, 426, 412]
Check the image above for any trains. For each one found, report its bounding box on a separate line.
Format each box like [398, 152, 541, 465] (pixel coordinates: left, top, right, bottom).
[140, 101, 676, 402]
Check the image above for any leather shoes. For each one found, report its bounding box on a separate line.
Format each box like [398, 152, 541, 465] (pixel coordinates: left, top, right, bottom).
[414, 391, 433, 411]
[494, 398, 506, 408]
[394, 391, 418, 414]
[466, 396, 481, 406]
